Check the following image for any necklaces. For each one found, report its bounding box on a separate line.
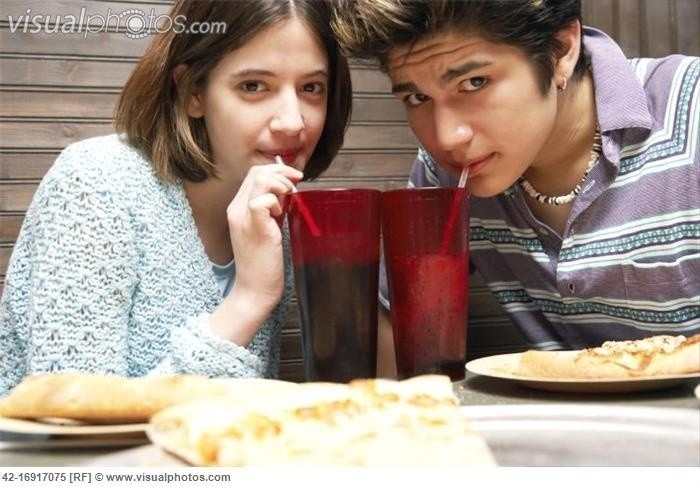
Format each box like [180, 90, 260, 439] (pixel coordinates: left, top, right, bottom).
[518, 123, 603, 208]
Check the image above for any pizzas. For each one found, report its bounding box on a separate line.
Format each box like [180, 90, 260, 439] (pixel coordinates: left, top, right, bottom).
[1, 371, 160, 426]
[149, 373, 499, 467]
[511, 335, 700, 381]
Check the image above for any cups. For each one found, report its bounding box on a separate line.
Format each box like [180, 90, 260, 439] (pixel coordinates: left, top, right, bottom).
[383, 185, 470, 384]
[285, 188, 383, 384]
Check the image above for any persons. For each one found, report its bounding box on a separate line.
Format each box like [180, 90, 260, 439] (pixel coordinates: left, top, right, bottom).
[0, 0, 357, 397]
[328, 0, 700, 380]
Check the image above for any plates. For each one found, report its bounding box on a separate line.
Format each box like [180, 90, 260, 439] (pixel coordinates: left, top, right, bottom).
[1, 375, 296, 438]
[462, 349, 700, 395]
